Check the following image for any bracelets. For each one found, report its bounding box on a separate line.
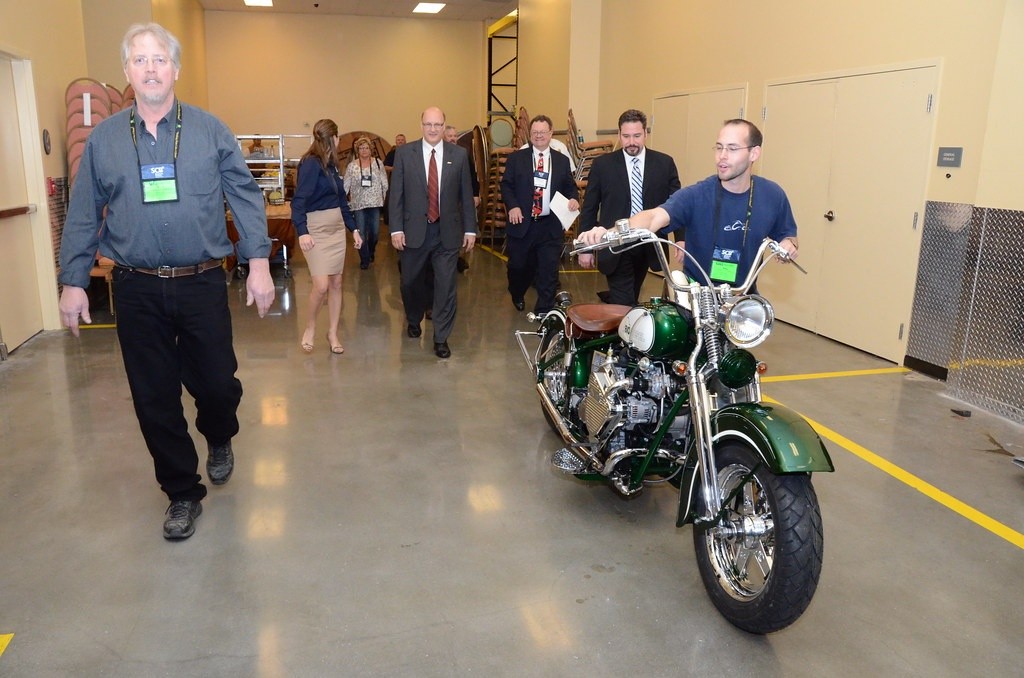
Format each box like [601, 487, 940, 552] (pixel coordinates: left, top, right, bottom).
[353, 229, 360, 232]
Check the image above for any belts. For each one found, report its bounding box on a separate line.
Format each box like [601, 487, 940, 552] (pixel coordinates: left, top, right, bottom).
[428, 219, 440, 223]
[113, 259, 223, 278]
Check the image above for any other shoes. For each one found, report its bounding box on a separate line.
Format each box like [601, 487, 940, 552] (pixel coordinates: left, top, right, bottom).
[327, 332, 344, 354]
[512, 293, 525, 311]
[300, 327, 316, 353]
[425, 309, 432, 320]
[360, 254, 375, 269]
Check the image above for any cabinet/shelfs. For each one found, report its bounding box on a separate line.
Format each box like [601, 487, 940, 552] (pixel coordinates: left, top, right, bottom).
[284, 134, 316, 162]
[234, 134, 285, 201]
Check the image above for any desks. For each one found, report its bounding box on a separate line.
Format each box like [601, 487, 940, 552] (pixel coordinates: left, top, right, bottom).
[228, 199, 293, 266]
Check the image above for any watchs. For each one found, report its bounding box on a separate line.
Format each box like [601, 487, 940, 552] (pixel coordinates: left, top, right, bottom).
[782, 238, 797, 249]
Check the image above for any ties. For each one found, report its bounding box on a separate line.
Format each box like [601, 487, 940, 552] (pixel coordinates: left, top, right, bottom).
[428, 149, 439, 223]
[631, 158, 643, 218]
[532, 153, 544, 222]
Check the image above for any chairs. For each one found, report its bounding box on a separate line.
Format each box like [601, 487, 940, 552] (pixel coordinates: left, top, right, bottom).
[62, 75, 136, 316]
[480, 107, 615, 267]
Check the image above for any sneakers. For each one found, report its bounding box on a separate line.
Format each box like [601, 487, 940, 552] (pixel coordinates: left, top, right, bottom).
[164, 498, 203, 539]
[206, 436, 234, 485]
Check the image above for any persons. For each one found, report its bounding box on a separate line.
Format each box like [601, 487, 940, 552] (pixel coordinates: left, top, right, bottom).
[577, 118, 797, 371]
[56, 21, 274, 539]
[580, 109, 686, 308]
[388, 106, 478, 358]
[243, 134, 268, 178]
[292, 119, 364, 354]
[343, 125, 479, 274]
[502, 115, 580, 322]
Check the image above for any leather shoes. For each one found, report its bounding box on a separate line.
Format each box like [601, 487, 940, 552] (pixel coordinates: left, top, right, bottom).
[407, 322, 422, 338]
[434, 338, 451, 359]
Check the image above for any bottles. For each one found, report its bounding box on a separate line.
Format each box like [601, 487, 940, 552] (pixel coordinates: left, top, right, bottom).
[262, 144, 275, 159]
[578, 129, 584, 144]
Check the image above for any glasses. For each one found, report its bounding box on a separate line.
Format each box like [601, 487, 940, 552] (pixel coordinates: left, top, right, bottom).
[134, 54, 177, 67]
[530, 130, 551, 136]
[422, 122, 444, 129]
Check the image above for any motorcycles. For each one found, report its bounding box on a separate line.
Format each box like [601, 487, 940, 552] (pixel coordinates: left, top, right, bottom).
[514, 218, 834, 634]
